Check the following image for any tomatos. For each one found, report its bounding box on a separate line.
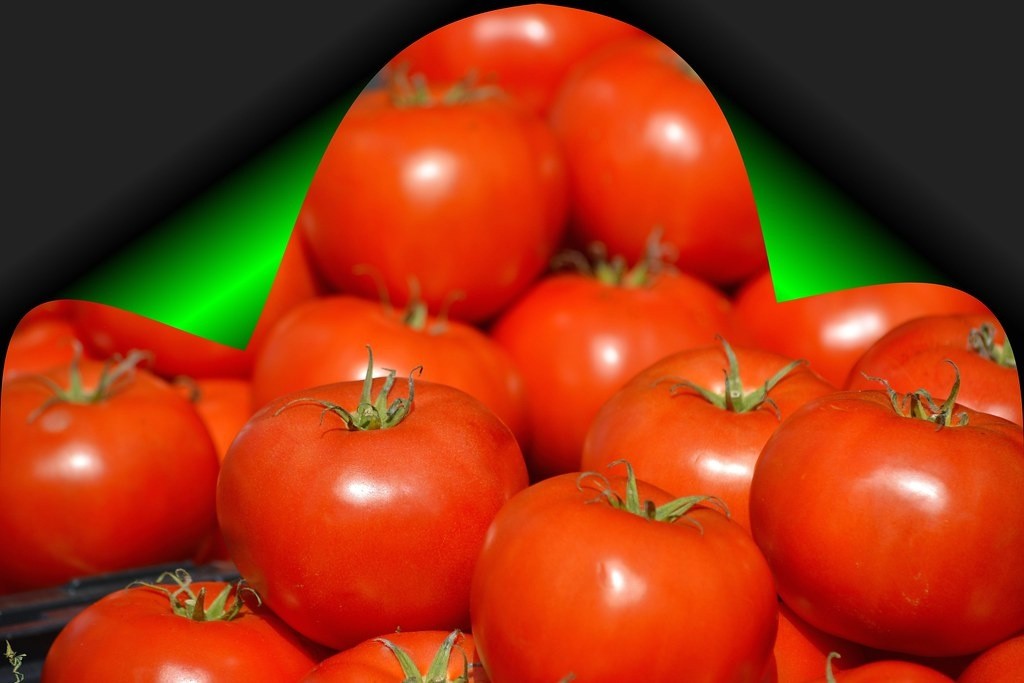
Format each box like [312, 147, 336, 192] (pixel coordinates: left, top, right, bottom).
[0, 4, 1024, 683]
